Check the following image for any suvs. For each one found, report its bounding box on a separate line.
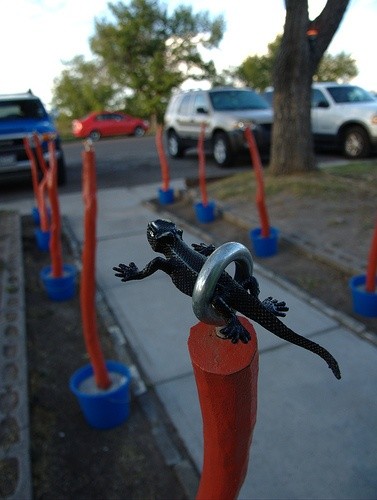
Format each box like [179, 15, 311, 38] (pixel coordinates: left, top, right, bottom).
[265, 83, 377, 158]
[0, 89, 66, 191]
[165, 89, 274, 166]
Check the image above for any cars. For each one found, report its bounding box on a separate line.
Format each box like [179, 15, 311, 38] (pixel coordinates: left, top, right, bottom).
[72, 111, 150, 141]
